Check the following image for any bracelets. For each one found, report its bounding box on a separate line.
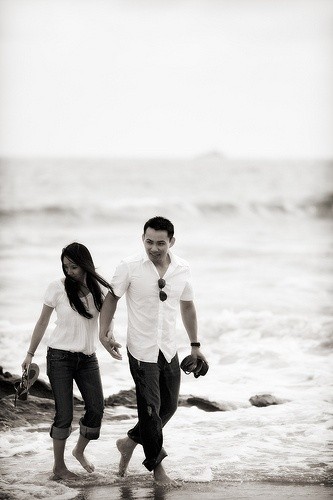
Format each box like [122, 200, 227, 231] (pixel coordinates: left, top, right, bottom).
[99, 216, 209, 487]
[28, 352, 34, 356]
[191, 342, 200, 347]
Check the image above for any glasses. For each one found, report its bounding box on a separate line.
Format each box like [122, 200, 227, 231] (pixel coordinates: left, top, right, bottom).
[158, 278, 167, 302]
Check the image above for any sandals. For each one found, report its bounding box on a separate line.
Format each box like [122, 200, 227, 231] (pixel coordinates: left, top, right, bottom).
[179, 355, 209, 378]
[13, 363, 40, 407]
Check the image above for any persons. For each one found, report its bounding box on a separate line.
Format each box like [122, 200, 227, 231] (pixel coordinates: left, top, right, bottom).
[22, 243, 122, 480]
